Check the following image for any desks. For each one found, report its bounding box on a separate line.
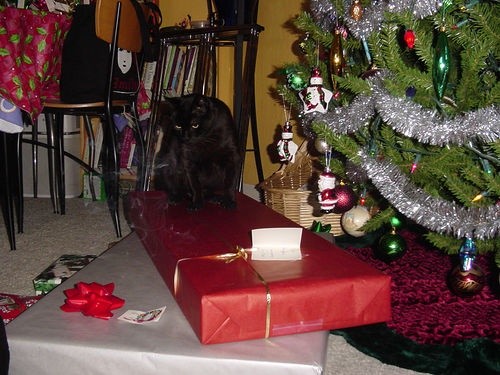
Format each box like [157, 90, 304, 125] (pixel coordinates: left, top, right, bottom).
[0, 7, 151, 250]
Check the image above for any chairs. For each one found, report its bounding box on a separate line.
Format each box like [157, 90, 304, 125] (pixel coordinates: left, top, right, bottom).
[43, 0, 150, 238]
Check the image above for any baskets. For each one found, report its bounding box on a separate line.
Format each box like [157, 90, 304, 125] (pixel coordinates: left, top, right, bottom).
[258, 137, 346, 236]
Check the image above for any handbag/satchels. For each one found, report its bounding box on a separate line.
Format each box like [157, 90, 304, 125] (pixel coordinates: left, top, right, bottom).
[60, 0, 162, 104]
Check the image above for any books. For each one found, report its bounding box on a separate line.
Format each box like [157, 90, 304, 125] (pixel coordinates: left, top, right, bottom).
[116, 42, 215, 169]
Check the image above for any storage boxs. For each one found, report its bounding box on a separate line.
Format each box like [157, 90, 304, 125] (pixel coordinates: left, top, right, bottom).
[33, 254, 97, 296]
[4, 231, 329, 375]
[127, 190, 392, 345]
